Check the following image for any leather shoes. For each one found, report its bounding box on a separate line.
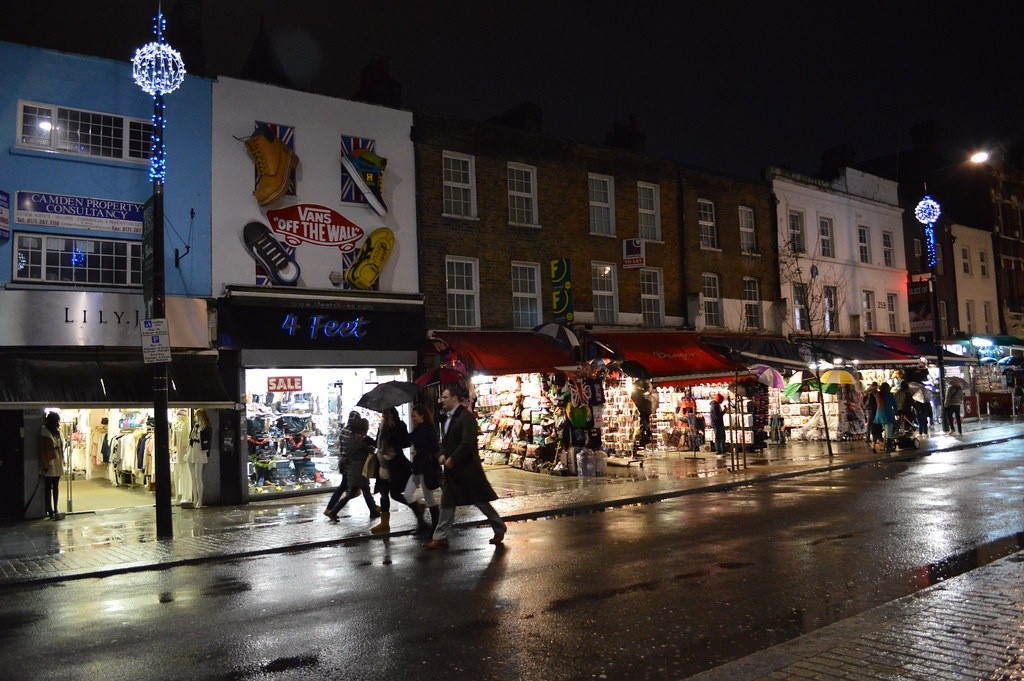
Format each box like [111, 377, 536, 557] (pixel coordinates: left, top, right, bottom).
[489, 525, 507, 544]
[422, 539, 450, 548]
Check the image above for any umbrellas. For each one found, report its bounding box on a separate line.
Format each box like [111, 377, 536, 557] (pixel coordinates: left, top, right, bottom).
[531, 322, 659, 415]
[998, 355, 1024, 367]
[789, 368, 824, 384]
[820, 370, 857, 384]
[746, 364, 785, 389]
[356, 380, 424, 414]
[908, 382, 933, 403]
[413, 367, 466, 403]
[935, 376, 974, 389]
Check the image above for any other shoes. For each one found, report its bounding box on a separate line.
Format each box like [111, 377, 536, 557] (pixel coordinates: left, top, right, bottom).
[922, 434, 927, 437]
[328, 510, 340, 522]
[916, 434, 922, 437]
[370, 512, 381, 519]
[323, 509, 339, 518]
[948, 429, 955, 435]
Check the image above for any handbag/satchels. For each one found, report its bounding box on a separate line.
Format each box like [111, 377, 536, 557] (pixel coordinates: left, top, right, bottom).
[362, 453, 378, 478]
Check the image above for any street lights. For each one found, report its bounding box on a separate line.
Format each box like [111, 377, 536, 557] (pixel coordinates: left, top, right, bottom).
[913, 148, 990, 432]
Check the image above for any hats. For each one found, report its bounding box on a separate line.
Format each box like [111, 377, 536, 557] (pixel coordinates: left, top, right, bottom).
[715, 393, 724, 403]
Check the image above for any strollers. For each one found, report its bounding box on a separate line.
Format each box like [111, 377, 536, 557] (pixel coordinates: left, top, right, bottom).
[882, 410, 920, 451]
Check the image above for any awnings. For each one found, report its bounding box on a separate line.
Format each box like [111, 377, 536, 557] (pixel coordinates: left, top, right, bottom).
[427, 328, 1024, 388]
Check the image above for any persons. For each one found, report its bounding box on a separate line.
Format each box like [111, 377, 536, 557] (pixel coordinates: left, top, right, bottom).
[709, 394, 728, 454]
[422, 387, 507, 549]
[327, 419, 382, 522]
[39, 411, 66, 519]
[186, 408, 213, 508]
[364, 407, 426, 536]
[861, 379, 964, 453]
[403, 403, 442, 538]
[873, 382, 899, 453]
[324, 411, 382, 520]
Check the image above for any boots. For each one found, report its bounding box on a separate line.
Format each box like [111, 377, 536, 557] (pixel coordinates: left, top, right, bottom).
[410, 500, 429, 536]
[884, 437, 895, 452]
[371, 513, 390, 533]
[426, 504, 439, 539]
